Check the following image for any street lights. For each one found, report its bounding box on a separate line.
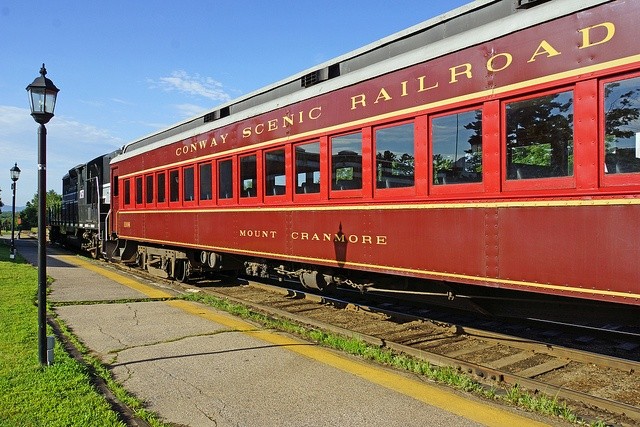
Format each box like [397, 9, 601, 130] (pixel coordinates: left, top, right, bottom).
[26, 63, 60, 364]
[9, 162, 22, 262]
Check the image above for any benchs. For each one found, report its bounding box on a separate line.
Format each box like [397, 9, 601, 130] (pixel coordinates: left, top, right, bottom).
[207, 193, 211, 199]
[380, 176, 413, 187]
[514, 162, 557, 179]
[603, 152, 640, 173]
[225, 191, 231, 198]
[302, 182, 320, 194]
[273, 185, 286, 195]
[336, 179, 362, 189]
[247, 187, 256, 197]
[438, 167, 479, 184]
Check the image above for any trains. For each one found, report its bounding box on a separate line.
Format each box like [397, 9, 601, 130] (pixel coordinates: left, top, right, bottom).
[47, 0, 640, 325]
